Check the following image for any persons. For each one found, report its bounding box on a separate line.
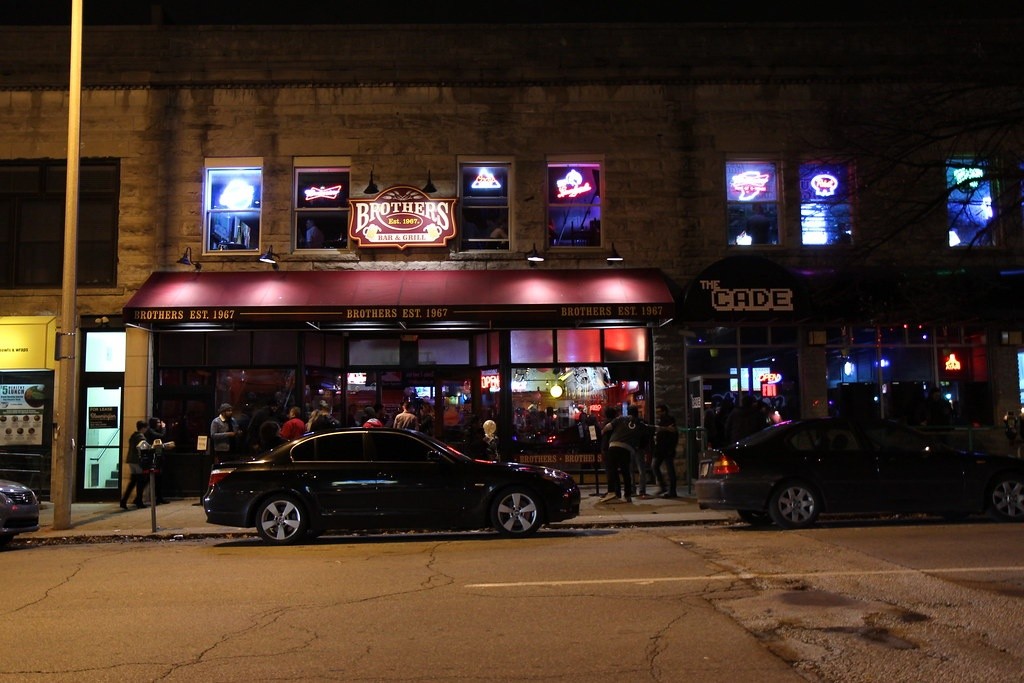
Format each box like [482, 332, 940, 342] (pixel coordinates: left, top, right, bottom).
[462, 211, 509, 250]
[702, 392, 787, 447]
[120, 418, 170, 510]
[463, 416, 498, 459]
[600, 403, 680, 502]
[211, 393, 341, 460]
[304, 218, 324, 248]
[347, 397, 434, 439]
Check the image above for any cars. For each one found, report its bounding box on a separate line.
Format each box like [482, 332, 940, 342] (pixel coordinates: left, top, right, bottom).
[201, 426, 582, 547]
[0, 480, 40, 539]
[695, 415, 1024, 529]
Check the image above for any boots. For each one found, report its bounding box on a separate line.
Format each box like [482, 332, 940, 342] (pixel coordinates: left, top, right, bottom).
[656, 470, 668, 495]
[662, 478, 676, 500]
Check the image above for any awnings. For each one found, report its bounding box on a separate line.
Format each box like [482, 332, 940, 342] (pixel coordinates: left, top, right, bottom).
[122, 270, 675, 320]
[684, 254, 1024, 333]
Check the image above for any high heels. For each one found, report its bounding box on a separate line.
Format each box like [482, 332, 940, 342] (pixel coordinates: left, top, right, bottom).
[133, 499, 137, 504]
[155, 497, 169, 505]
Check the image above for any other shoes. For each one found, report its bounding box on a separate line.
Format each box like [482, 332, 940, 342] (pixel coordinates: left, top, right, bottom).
[641, 492, 653, 499]
[623, 495, 632, 503]
[136, 500, 147, 508]
[120, 501, 128, 510]
[617, 494, 621, 498]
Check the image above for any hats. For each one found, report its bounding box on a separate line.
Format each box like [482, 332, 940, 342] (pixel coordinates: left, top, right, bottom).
[217, 403, 232, 414]
